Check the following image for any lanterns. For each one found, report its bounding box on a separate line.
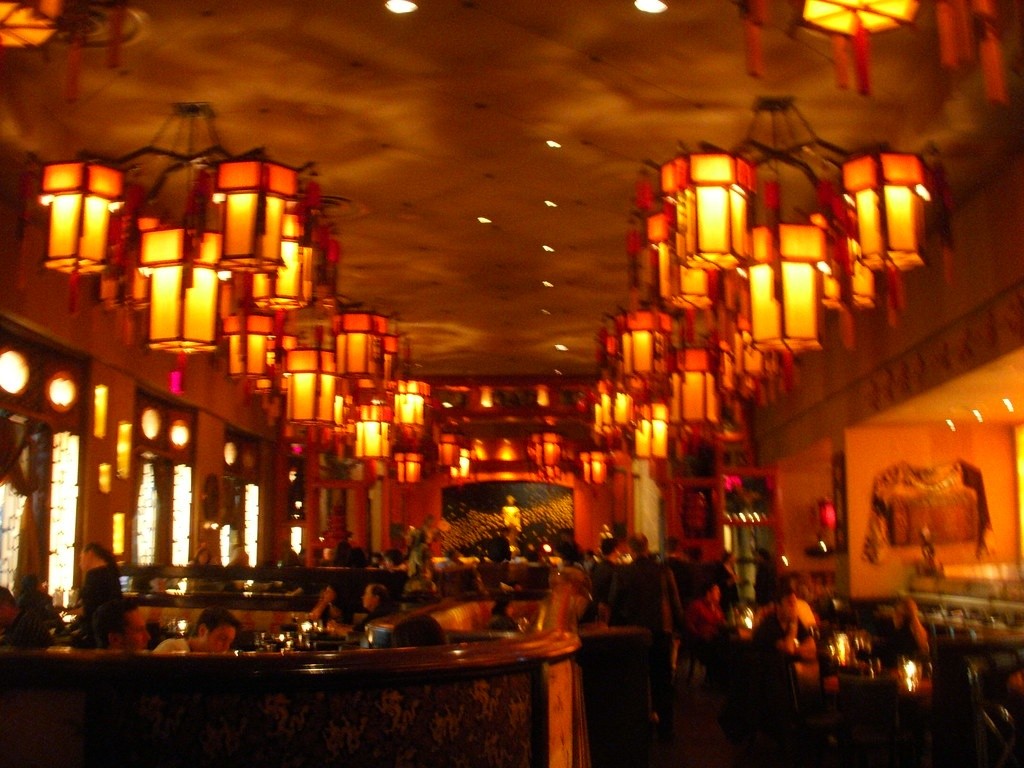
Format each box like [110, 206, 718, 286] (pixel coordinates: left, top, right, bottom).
[1, 0, 939, 481]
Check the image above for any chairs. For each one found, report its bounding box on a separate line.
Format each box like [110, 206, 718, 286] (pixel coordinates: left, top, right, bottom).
[675, 607, 1024, 768]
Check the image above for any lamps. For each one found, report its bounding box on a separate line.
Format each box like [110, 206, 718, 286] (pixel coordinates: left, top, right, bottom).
[0, 0, 1011, 490]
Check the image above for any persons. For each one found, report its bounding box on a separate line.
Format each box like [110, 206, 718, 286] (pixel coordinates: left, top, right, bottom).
[1, 520, 931, 768]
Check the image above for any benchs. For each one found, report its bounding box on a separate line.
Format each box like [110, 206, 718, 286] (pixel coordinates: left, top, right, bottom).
[0, 553, 594, 768]
